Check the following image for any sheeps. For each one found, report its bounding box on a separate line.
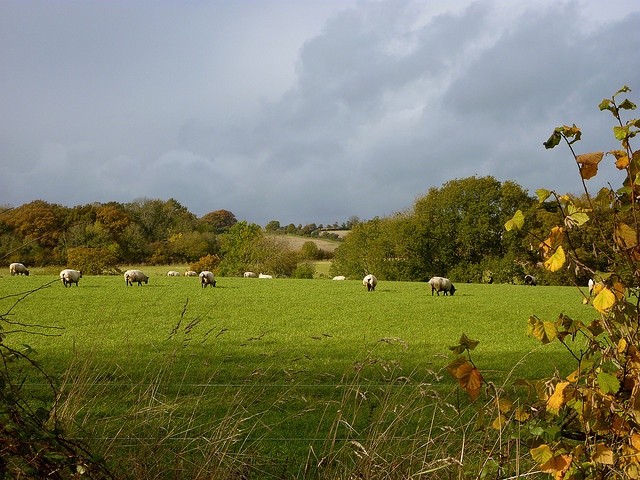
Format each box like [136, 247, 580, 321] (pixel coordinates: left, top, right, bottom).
[428, 276, 457, 296]
[124, 270, 149, 286]
[244, 272, 254, 278]
[199, 271, 216, 288]
[185, 271, 197, 276]
[333, 276, 345, 280]
[259, 272, 272, 278]
[60, 269, 82, 288]
[525, 275, 537, 286]
[9, 263, 29, 276]
[363, 274, 378, 291]
[167, 271, 180, 276]
